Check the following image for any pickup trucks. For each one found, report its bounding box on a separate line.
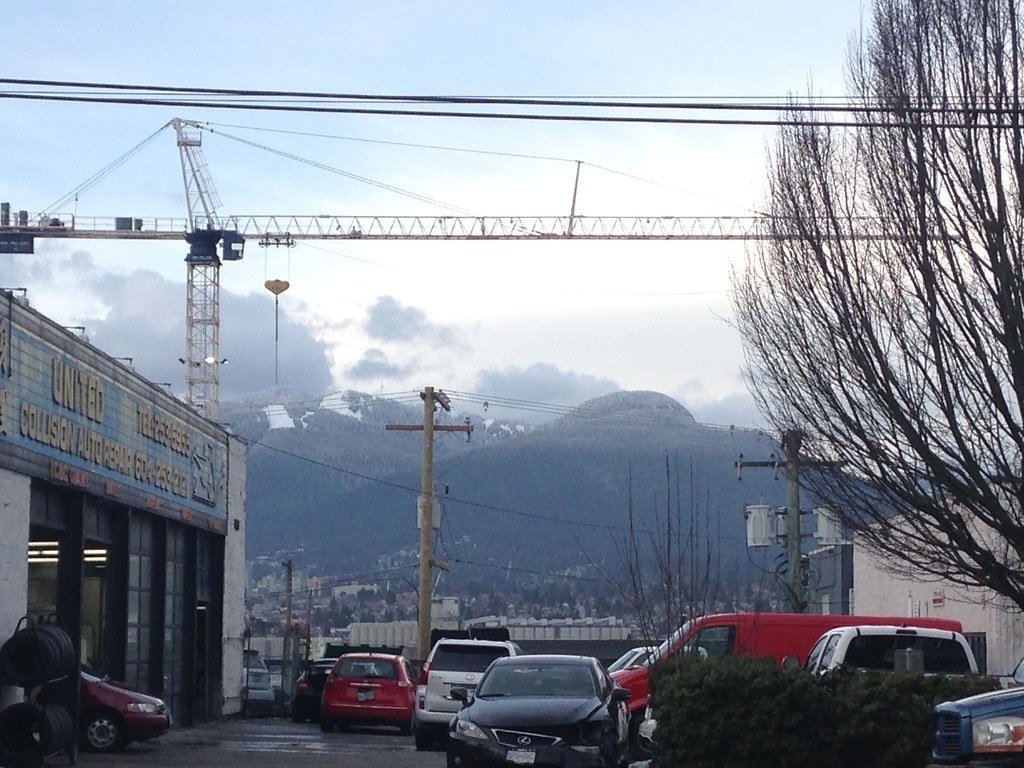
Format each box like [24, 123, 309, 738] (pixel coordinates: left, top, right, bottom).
[780, 624, 1015, 691]
[606, 647, 708, 673]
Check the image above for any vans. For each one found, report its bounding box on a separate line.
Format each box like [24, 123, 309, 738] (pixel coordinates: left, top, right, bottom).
[609, 612, 962, 755]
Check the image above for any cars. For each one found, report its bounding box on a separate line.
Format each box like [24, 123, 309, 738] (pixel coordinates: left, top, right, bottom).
[79, 671, 175, 753]
[931, 686, 1024, 768]
[322, 652, 418, 732]
[448, 653, 633, 768]
[292, 663, 336, 723]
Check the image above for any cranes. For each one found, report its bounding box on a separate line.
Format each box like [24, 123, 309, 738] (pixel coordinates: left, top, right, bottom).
[0, 115, 964, 422]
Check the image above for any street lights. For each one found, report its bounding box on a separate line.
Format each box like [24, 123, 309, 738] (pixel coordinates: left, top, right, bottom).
[417, 559, 453, 661]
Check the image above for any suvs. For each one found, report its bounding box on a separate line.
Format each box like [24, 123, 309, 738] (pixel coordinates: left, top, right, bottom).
[243, 648, 276, 712]
[414, 637, 527, 745]
